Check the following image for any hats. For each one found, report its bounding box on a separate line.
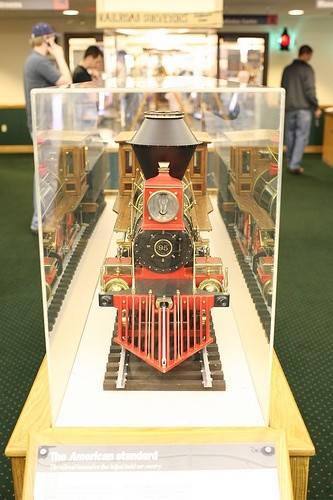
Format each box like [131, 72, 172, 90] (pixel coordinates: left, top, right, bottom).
[31, 22, 60, 38]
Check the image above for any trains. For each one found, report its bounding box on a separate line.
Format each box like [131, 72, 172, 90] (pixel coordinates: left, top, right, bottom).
[97, 94, 231, 375]
[37, 138, 109, 300]
[210, 139, 280, 315]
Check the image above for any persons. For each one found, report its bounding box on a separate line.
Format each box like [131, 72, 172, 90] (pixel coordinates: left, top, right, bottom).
[280, 44, 322, 174]
[71, 46, 104, 82]
[23, 21, 72, 234]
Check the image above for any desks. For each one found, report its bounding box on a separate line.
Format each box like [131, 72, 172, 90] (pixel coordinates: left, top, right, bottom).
[5, 188, 316, 500]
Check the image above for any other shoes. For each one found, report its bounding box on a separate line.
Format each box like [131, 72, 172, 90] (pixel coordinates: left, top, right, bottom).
[285, 166, 304, 175]
[30, 228, 38, 234]
